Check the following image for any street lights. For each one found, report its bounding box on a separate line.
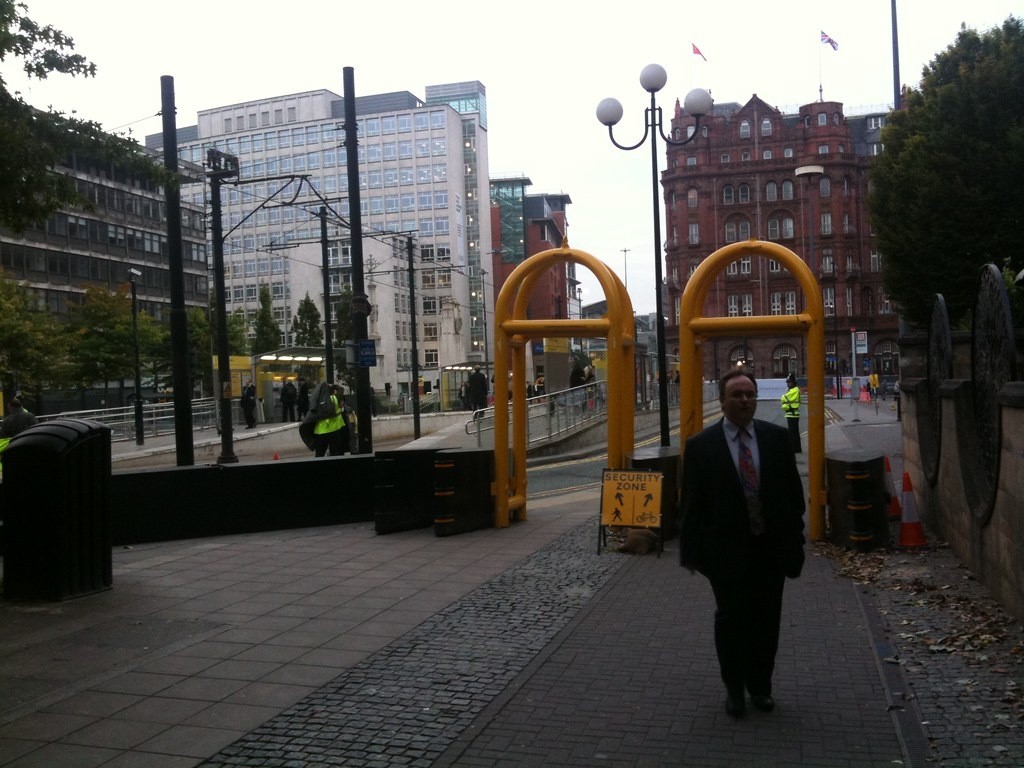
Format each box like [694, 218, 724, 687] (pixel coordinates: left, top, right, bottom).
[794, 165, 826, 274]
[595, 63, 712, 448]
[620, 250, 631, 290]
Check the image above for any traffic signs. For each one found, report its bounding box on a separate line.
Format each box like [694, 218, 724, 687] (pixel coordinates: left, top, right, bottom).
[599, 468, 664, 529]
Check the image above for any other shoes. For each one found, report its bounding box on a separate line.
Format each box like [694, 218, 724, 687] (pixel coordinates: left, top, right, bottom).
[245, 425, 252, 429]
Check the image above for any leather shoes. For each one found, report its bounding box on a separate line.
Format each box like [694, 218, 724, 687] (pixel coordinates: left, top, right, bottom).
[752, 691, 775, 712]
[725, 689, 745, 715]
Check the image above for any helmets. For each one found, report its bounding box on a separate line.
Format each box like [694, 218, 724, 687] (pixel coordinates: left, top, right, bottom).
[785, 373, 796, 383]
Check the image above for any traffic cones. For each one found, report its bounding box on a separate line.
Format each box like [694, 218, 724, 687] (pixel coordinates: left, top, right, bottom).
[892, 469, 936, 551]
[883, 455, 902, 522]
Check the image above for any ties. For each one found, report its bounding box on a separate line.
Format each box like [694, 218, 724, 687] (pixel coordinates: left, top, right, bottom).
[738, 428, 761, 537]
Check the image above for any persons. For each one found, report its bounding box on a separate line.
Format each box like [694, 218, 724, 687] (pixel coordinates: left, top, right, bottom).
[240, 362, 594, 458]
[677, 370, 806, 718]
[781, 374, 802, 454]
[0, 398, 41, 438]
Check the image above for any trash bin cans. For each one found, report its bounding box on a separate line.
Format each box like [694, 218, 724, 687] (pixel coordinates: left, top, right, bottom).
[252, 397, 265, 424]
[403, 396, 413, 413]
[826, 448, 890, 550]
[0, 418, 114, 606]
